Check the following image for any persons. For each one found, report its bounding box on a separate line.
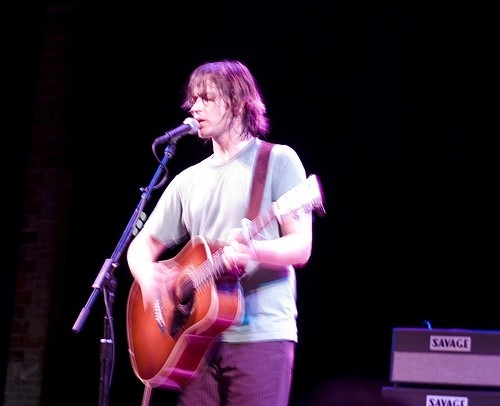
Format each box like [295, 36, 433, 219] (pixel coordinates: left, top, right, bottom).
[126, 61, 312, 405]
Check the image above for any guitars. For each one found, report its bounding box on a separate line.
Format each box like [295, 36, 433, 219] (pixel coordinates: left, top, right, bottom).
[126, 173, 325, 389]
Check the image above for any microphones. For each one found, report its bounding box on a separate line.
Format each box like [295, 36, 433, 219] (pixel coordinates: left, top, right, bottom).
[156, 119, 200, 144]
